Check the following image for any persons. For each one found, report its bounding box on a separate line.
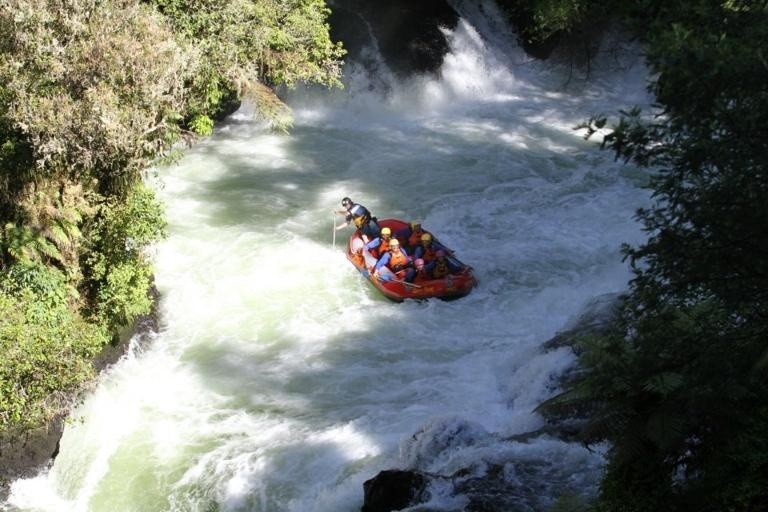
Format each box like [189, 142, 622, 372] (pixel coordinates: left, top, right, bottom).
[371, 238, 413, 278]
[411, 232, 453, 265]
[335, 197, 380, 237]
[355, 227, 399, 258]
[416, 249, 467, 280]
[391, 219, 440, 255]
[399, 255, 440, 283]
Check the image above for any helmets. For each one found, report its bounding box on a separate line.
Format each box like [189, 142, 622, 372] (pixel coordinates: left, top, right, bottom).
[414, 258, 424, 266]
[421, 232, 432, 241]
[381, 227, 391, 235]
[435, 249, 446, 258]
[342, 198, 351, 206]
[389, 238, 400, 249]
[411, 219, 421, 230]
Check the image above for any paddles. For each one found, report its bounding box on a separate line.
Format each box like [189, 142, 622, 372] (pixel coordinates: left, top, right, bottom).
[379, 274, 421, 288]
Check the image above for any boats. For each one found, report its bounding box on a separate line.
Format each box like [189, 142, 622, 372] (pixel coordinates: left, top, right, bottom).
[344, 217, 478, 304]
